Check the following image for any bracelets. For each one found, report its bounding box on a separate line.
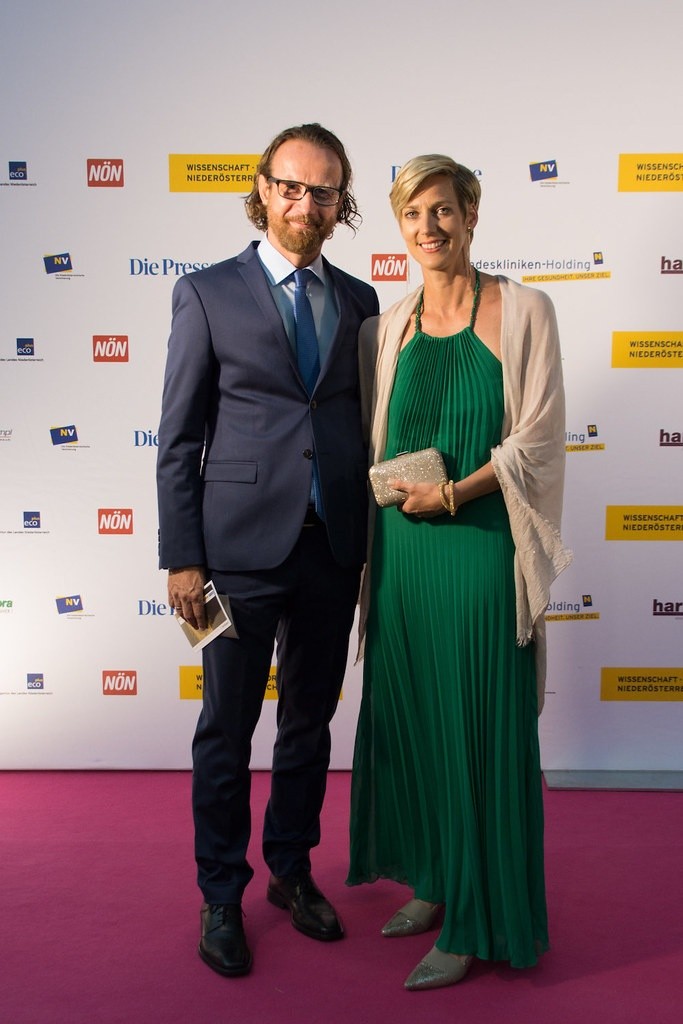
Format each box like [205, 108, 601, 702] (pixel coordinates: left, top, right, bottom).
[448, 479, 456, 516]
[438, 481, 458, 511]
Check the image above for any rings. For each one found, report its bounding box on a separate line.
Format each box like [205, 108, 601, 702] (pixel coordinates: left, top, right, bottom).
[174, 606, 182, 610]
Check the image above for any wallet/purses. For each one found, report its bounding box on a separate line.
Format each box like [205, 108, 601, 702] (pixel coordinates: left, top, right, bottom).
[369, 447, 448, 508]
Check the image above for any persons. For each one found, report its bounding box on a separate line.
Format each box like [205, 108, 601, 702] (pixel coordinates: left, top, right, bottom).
[155, 123, 382, 980]
[343, 155, 567, 990]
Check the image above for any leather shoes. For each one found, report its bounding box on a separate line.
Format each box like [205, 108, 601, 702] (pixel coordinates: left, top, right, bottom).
[267, 873, 345, 942]
[198, 901, 253, 977]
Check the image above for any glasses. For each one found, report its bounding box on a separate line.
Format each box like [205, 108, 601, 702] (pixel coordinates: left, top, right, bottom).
[268, 176, 343, 206]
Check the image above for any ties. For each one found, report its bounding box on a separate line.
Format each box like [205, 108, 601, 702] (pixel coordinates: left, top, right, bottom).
[293, 269, 316, 398]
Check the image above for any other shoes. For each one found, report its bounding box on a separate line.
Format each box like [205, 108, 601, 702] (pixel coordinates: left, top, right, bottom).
[405, 946, 474, 990]
[381, 898, 442, 937]
